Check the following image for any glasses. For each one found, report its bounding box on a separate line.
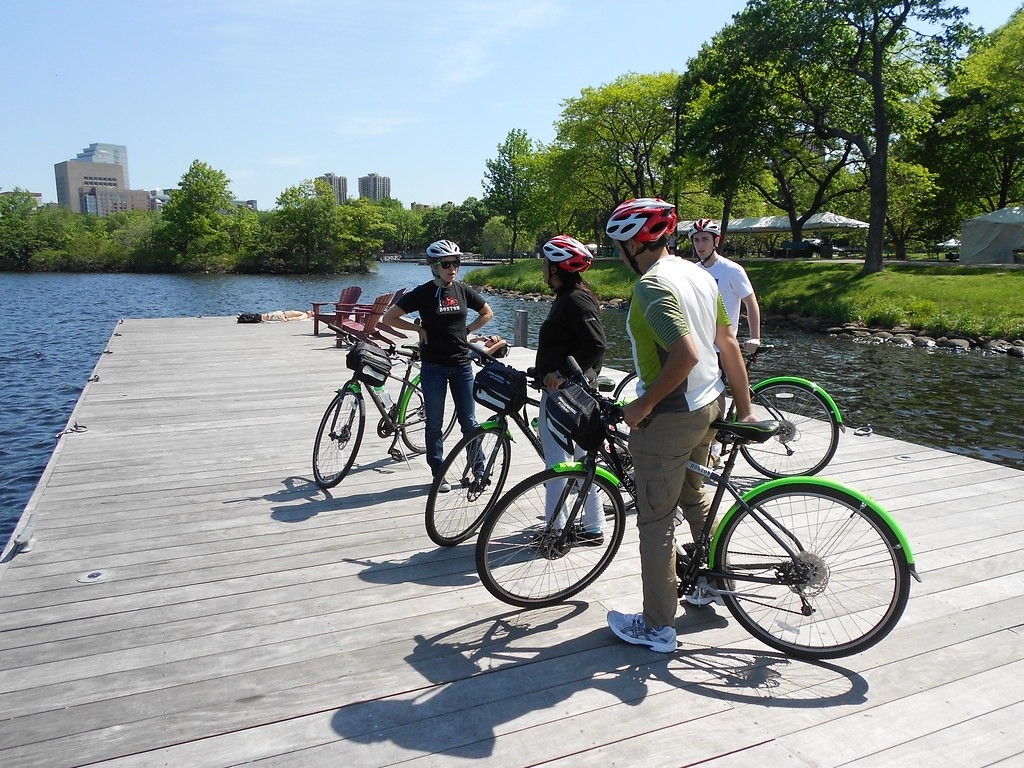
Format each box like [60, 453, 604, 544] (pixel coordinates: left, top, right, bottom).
[441, 260, 460, 269]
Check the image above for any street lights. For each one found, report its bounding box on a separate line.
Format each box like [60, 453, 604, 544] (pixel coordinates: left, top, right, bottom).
[665, 106, 680, 249]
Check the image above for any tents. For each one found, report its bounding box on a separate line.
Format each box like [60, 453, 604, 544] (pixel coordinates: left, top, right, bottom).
[673, 211, 870, 259]
[939, 238, 961, 247]
[958, 205, 1024, 266]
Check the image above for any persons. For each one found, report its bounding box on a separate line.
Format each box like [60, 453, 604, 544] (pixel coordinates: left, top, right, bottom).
[382, 239, 494, 493]
[606, 197, 757, 653]
[687, 217, 761, 471]
[535, 235, 607, 551]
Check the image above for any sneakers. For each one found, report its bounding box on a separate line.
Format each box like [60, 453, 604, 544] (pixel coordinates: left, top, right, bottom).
[686, 588, 727, 606]
[607, 611, 678, 653]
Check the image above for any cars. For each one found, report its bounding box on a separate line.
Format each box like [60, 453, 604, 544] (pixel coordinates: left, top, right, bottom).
[936, 239, 961, 250]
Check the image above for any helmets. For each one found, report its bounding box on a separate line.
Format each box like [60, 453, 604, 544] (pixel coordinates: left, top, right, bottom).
[543, 235, 594, 273]
[688, 218, 721, 242]
[426, 239, 463, 264]
[605, 198, 677, 242]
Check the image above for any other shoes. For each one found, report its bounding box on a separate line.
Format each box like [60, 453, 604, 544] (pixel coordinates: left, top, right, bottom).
[433, 477, 451, 492]
[570, 528, 604, 546]
[476, 476, 488, 491]
[713, 455, 720, 466]
[533, 531, 568, 544]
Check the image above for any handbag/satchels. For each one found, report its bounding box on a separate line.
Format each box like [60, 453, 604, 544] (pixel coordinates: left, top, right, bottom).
[238, 313, 262, 323]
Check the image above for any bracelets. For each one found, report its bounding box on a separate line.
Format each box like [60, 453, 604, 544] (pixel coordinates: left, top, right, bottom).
[465, 326, 470, 335]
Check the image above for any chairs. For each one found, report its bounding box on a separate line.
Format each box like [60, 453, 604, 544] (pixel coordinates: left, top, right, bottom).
[310, 285, 408, 349]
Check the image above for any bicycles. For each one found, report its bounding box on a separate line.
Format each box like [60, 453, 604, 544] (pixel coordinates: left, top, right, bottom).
[475, 354, 922, 662]
[313, 322, 458, 490]
[613, 343, 845, 480]
[425, 341, 633, 548]
